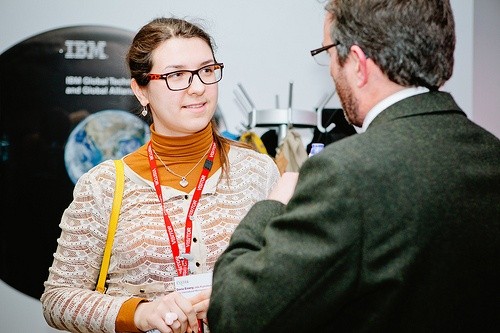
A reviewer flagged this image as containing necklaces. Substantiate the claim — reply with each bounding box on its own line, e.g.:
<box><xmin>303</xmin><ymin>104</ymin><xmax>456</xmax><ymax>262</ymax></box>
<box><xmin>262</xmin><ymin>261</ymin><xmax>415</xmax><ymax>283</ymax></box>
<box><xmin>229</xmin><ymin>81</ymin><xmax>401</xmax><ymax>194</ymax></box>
<box><xmin>152</xmin><ymin>141</ymin><xmax>213</xmax><ymax>188</ymax></box>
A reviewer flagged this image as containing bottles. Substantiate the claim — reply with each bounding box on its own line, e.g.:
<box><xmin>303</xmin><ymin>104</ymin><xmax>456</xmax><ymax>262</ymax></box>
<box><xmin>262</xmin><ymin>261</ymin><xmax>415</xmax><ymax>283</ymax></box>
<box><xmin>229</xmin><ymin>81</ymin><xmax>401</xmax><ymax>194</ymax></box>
<box><xmin>308</xmin><ymin>144</ymin><xmax>323</xmax><ymax>158</ymax></box>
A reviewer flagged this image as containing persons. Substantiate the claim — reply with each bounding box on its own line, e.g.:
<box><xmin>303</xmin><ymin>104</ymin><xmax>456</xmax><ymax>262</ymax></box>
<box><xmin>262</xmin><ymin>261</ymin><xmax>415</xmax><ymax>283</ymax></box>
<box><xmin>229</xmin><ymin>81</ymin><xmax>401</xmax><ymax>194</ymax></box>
<box><xmin>206</xmin><ymin>0</ymin><xmax>500</xmax><ymax>333</ymax></box>
<box><xmin>40</xmin><ymin>18</ymin><xmax>281</xmax><ymax>333</ymax></box>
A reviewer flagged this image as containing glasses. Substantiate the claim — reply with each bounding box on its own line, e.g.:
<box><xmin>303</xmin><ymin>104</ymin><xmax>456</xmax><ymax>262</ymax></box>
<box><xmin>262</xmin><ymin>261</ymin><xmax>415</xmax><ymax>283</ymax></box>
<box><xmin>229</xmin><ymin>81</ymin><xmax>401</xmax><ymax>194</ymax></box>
<box><xmin>148</xmin><ymin>62</ymin><xmax>224</xmax><ymax>92</ymax></box>
<box><xmin>310</xmin><ymin>42</ymin><xmax>340</xmax><ymax>68</ymax></box>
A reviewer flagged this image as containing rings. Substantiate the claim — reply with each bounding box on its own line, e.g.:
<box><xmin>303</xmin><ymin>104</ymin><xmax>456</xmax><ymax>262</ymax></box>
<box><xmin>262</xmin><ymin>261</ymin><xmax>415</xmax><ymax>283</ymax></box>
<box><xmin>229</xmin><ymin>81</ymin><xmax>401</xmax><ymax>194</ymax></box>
<box><xmin>165</xmin><ymin>312</ymin><xmax>178</xmax><ymax>325</ymax></box>
